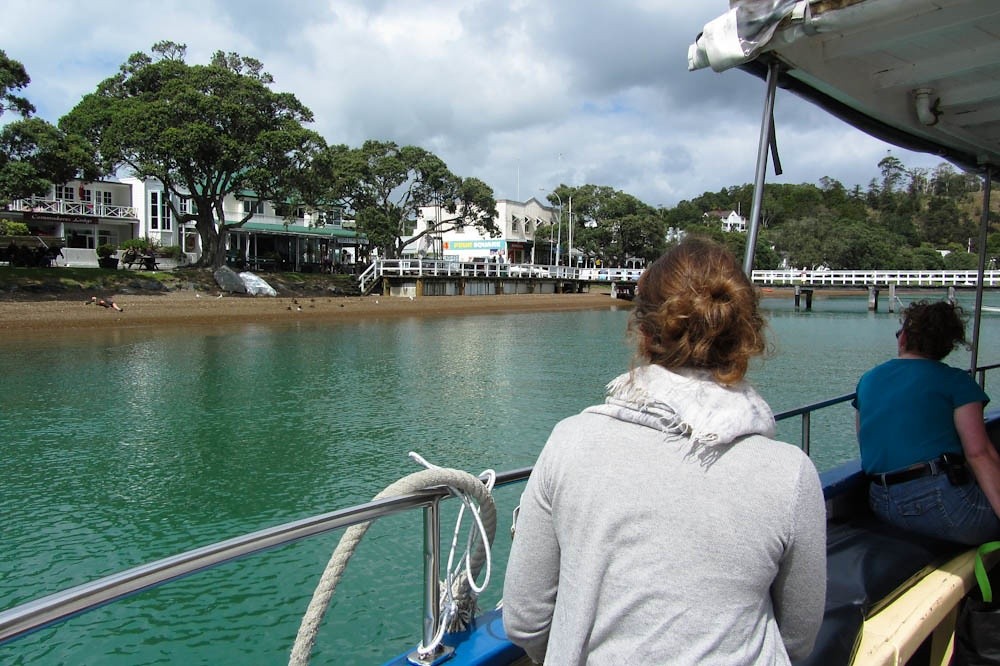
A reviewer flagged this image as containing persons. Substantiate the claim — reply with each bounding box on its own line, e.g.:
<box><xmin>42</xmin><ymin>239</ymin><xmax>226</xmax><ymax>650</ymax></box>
<box><xmin>121</xmin><ymin>245</ymin><xmax>158</xmax><ymax>271</ymax></box>
<box><xmin>500</xmin><ymin>238</ymin><xmax>827</xmax><ymax>665</ymax></box>
<box><xmin>340</xmin><ymin>246</ymin><xmax>648</xmax><ymax>286</ymax></box>
<box><xmin>850</xmin><ymin>296</ymin><xmax>1000</xmax><ymax>552</ymax></box>
<box><xmin>6</xmin><ymin>237</ymin><xmax>53</xmax><ymax>267</ymax></box>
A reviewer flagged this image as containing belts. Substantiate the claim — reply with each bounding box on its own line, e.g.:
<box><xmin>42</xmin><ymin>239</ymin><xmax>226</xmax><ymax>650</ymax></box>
<box><xmin>873</xmin><ymin>452</ymin><xmax>954</xmax><ymax>485</ymax></box>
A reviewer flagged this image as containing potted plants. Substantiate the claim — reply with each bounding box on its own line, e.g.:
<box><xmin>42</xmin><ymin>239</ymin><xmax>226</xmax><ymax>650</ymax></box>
<box><xmin>96</xmin><ymin>244</ymin><xmax>119</xmax><ymax>269</ymax></box>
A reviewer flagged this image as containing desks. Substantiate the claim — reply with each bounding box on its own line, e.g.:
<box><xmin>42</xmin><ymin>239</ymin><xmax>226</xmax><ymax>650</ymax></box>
<box><xmin>127</xmin><ymin>255</ymin><xmax>152</xmax><ymax>270</ymax></box>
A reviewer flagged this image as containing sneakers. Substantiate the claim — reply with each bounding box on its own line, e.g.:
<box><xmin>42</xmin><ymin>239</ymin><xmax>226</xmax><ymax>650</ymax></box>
<box><xmin>962</xmin><ymin>601</ymin><xmax>1000</xmax><ymax>660</ymax></box>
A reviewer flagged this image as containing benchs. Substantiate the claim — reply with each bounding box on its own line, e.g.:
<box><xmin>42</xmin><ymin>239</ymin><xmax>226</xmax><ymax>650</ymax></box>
<box><xmin>127</xmin><ymin>262</ymin><xmax>160</xmax><ymax>270</ymax></box>
<box><xmin>850</xmin><ymin>548</ymin><xmax>1000</xmax><ymax>666</ymax></box>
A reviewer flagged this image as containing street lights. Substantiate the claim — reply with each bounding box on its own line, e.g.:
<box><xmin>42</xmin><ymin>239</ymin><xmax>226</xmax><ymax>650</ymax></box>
<box><xmin>539</xmin><ymin>188</ymin><xmax>561</xmax><ymax>266</ymax></box>
